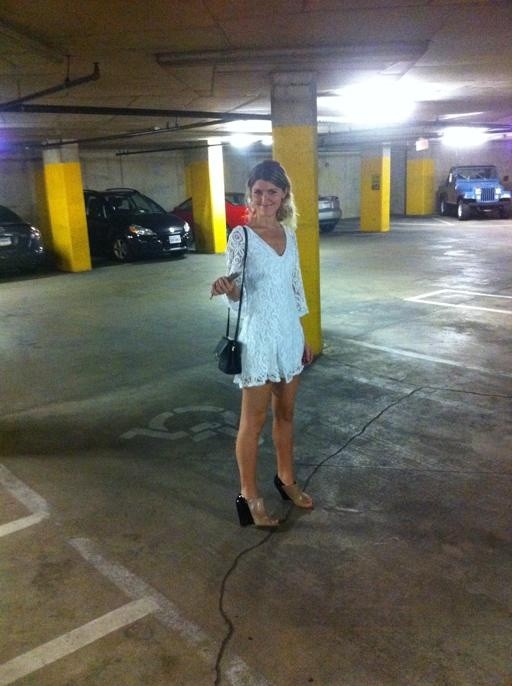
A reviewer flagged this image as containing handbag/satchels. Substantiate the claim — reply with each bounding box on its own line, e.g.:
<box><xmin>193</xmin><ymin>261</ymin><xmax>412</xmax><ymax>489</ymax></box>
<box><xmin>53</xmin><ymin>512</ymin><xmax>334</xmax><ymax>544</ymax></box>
<box><xmin>213</xmin><ymin>336</ymin><xmax>242</xmax><ymax>375</ymax></box>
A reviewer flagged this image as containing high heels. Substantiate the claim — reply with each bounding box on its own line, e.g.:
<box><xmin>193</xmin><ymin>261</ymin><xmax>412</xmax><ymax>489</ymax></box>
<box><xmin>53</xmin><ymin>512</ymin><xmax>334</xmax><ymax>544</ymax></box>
<box><xmin>236</xmin><ymin>493</ymin><xmax>282</xmax><ymax>528</ymax></box>
<box><xmin>274</xmin><ymin>473</ymin><xmax>314</xmax><ymax>509</ymax></box>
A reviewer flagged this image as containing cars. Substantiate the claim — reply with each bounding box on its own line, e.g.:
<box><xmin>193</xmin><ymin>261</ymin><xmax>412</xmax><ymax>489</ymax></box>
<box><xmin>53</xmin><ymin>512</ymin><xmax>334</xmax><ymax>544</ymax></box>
<box><xmin>317</xmin><ymin>193</ymin><xmax>341</xmax><ymax>232</ymax></box>
<box><xmin>167</xmin><ymin>190</ymin><xmax>254</xmax><ymax>238</ymax></box>
<box><xmin>0</xmin><ymin>204</ymin><xmax>47</xmax><ymax>275</ymax></box>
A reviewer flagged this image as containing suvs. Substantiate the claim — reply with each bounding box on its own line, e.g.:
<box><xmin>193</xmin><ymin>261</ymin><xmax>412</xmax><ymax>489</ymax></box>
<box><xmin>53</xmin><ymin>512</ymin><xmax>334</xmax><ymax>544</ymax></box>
<box><xmin>438</xmin><ymin>164</ymin><xmax>512</xmax><ymax>222</ymax></box>
<box><xmin>84</xmin><ymin>187</ymin><xmax>195</xmax><ymax>263</ymax></box>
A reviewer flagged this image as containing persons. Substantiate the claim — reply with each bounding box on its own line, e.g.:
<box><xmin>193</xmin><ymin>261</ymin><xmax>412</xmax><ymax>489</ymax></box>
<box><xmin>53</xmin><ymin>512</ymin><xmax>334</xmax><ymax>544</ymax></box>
<box><xmin>209</xmin><ymin>157</ymin><xmax>318</xmax><ymax>530</ymax></box>
